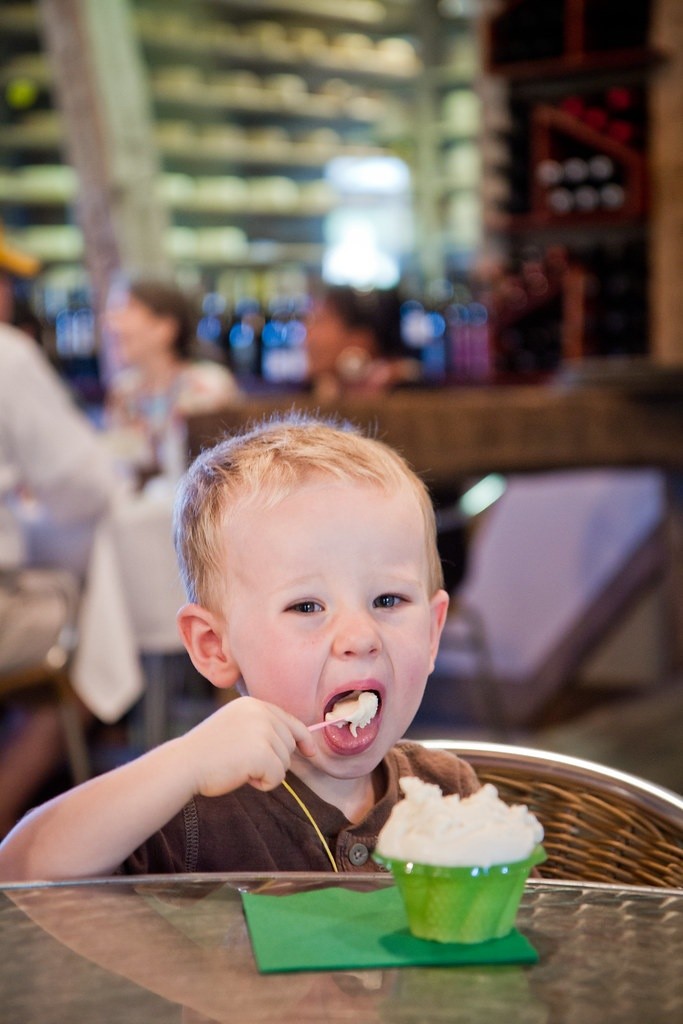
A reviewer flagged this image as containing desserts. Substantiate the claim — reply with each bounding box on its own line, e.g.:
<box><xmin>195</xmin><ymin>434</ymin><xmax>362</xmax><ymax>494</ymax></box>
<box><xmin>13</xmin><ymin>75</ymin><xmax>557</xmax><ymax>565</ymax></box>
<box><xmin>375</xmin><ymin>777</ymin><xmax>546</xmax><ymax>942</ymax></box>
<box><xmin>325</xmin><ymin>690</ymin><xmax>378</xmax><ymax>737</ymax></box>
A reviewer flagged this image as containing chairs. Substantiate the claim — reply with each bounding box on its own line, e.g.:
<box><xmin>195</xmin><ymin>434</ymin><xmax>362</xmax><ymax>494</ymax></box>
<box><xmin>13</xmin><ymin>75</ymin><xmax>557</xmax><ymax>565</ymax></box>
<box><xmin>411</xmin><ymin>736</ymin><xmax>683</xmax><ymax>889</ymax></box>
<box><xmin>420</xmin><ymin>471</ymin><xmax>669</xmax><ymax>727</ymax></box>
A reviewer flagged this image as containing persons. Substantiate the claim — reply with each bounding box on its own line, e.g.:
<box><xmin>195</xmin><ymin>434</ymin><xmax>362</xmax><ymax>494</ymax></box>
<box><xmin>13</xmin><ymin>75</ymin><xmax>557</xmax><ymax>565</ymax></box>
<box><xmin>0</xmin><ymin>232</ymin><xmax>475</xmax><ymax>828</ymax></box>
<box><xmin>3</xmin><ymin>408</ymin><xmax>547</xmax><ymax>880</ymax></box>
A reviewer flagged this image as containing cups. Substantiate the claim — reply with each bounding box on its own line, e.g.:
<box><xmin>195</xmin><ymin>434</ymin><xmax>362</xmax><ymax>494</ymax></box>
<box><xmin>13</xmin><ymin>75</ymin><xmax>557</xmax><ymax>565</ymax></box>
<box><xmin>372</xmin><ymin>841</ymin><xmax>546</xmax><ymax>946</ymax></box>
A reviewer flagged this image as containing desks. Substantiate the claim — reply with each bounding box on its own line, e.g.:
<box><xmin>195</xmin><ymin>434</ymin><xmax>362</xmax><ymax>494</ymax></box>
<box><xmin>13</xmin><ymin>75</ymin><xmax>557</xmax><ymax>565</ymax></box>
<box><xmin>0</xmin><ymin>871</ymin><xmax>683</xmax><ymax>1024</ymax></box>
